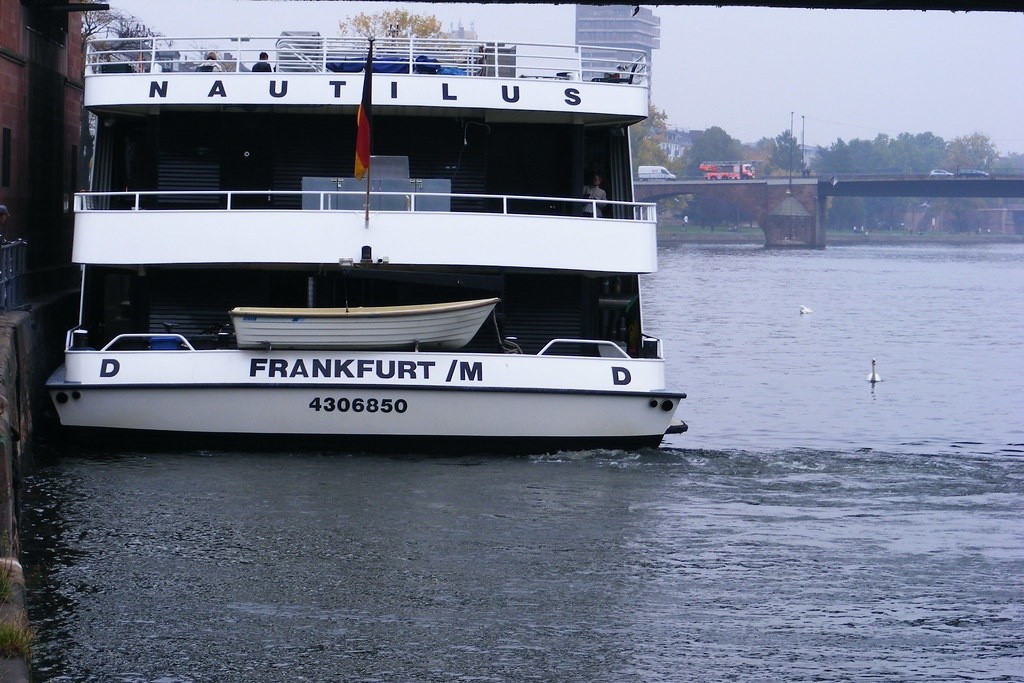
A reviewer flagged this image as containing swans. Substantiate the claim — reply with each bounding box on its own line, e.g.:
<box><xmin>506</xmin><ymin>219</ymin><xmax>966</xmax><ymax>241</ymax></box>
<box><xmin>867</xmin><ymin>358</ymin><xmax>881</xmax><ymax>381</ymax></box>
<box><xmin>799</xmin><ymin>305</ymin><xmax>812</xmax><ymax>313</ymax></box>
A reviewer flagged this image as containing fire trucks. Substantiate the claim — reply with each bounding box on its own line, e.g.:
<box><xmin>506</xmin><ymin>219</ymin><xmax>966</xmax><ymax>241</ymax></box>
<box><xmin>698</xmin><ymin>159</ymin><xmax>764</xmax><ymax>180</ymax></box>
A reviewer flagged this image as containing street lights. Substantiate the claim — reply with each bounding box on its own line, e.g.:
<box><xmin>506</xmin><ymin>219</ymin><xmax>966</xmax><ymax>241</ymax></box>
<box><xmin>801</xmin><ymin>115</ymin><xmax>805</xmax><ymax>178</ymax></box>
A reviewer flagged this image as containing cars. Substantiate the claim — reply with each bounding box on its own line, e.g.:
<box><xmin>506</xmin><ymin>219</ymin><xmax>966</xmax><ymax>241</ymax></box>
<box><xmin>955</xmin><ymin>169</ymin><xmax>989</xmax><ymax>178</ymax></box>
<box><xmin>929</xmin><ymin>169</ymin><xmax>954</xmax><ymax>178</ymax></box>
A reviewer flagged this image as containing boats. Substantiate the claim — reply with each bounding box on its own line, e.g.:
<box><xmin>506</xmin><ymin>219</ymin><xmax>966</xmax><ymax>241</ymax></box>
<box><xmin>41</xmin><ymin>38</ymin><xmax>690</xmax><ymax>452</ymax></box>
<box><xmin>226</xmin><ymin>296</ymin><xmax>503</xmax><ymax>350</ymax></box>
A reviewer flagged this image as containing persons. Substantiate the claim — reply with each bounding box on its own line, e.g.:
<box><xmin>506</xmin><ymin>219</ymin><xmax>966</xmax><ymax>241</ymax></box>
<box><xmin>201</xmin><ymin>52</ymin><xmax>222</xmax><ymax>72</ymax></box>
<box><xmin>153</xmin><ymin>63</ymin><xmax>162</xmax><ymax>72</ymax></box>
<box><xmin>582</xmin><ymin>174</ymin><xmax>607</xmax><ymax>217</ymax></box>
<box><xmin>354</xmin><ymin>45</ymin><xmax>372</xmax><ymax>181</ymax></box>
<box><xmin>252</xmin><ymin>52</ymin><xmax>272</xmax><ymax>72</ymax></box>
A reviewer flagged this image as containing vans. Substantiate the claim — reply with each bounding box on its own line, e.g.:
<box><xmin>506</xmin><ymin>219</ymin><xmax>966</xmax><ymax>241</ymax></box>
<box><xmin>637</xmin><ymin>165</ymin><xmax>676</xmax><ymax>181</ymax></box>
